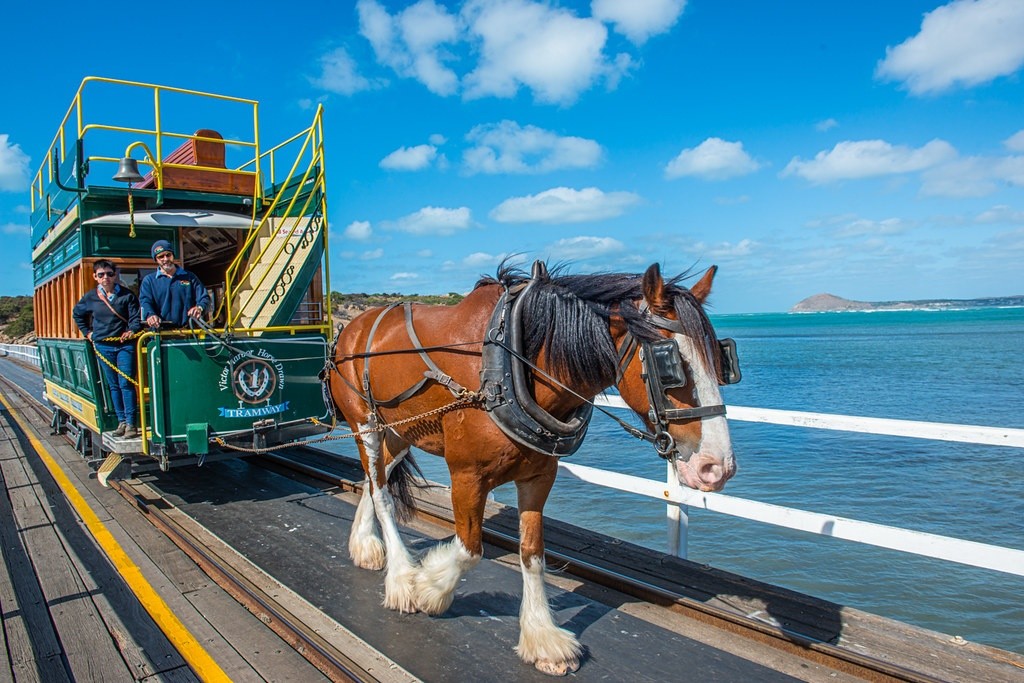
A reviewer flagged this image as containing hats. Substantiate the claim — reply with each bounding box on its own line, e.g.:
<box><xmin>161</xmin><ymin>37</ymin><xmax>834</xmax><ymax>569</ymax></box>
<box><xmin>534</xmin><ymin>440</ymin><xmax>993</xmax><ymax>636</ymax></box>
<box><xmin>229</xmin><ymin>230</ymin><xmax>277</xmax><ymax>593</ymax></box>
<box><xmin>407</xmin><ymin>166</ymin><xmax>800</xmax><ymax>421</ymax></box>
<box><xmin>150</xmin><ymin>239</ymin><xmax>174</xmax><ymax>262</ymax></box>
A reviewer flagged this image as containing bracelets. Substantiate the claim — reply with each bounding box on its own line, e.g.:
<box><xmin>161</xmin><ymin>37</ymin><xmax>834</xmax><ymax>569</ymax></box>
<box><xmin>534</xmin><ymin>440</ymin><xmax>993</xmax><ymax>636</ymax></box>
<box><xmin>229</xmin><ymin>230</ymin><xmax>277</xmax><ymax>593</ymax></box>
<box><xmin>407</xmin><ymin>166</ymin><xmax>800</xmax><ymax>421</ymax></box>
<box><xmin>198</xmin><ymin>306</ymin><xmax>203</xmax><ymax>311</ymax></box>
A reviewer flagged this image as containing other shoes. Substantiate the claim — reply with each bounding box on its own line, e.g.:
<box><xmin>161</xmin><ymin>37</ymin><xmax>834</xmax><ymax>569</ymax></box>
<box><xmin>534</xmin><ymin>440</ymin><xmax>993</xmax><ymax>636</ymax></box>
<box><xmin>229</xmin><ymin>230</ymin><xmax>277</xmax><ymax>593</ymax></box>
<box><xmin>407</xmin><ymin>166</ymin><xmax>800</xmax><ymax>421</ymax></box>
<box><xmin>122</xmin><ymin>425</ymin><xmax>137</xmax><ymax>439</ymax></box>
<box><xmin>112</xmin><ymin>422</ymin><xmax>126</xmax><ymax>437</ymax></box>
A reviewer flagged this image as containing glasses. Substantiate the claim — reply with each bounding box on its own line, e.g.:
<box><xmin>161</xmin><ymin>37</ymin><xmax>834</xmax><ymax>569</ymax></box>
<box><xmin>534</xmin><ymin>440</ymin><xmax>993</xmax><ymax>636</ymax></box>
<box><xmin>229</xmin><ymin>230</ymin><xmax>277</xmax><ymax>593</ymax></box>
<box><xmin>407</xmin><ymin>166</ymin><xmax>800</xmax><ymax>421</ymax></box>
<box><xmin>156</xmin><ymin>252</ymin><xmax>172</xmax><ymax>260</ymax></box>
<box><xmin>95</xmin><ymin>272</ymin><xmax>115</xmax><ymax>278</ymax></box>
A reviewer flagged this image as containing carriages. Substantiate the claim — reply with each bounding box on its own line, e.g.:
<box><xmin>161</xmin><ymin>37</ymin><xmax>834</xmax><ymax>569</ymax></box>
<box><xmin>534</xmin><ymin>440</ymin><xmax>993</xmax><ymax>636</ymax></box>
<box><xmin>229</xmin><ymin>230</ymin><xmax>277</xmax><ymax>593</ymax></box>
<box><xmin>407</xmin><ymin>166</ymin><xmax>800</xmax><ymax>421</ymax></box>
<box><xmin>27</xmin><ymin>74</ymin><xmax>741</xmax><ymax>678</ymax></box>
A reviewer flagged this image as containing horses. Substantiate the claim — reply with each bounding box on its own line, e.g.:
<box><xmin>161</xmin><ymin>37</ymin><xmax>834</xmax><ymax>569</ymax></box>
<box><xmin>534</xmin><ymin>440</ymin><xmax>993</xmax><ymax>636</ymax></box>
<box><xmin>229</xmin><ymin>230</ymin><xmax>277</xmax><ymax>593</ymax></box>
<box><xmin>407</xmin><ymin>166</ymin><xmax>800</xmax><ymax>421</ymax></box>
<box><xmin>328</xmin><ymin>264</ymin><xmax>737</xmax><ymax>678</ymax></box>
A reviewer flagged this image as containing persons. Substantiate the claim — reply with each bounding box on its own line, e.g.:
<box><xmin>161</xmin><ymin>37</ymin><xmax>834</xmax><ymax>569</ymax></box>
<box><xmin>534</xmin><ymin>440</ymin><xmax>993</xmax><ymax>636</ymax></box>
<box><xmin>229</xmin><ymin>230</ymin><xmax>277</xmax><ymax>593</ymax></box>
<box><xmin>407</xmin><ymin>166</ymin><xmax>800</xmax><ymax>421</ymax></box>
<box><xmin>139</xmin><ymin>239</ymin><xmax>212</xmax><ymax>339</ymax></box>
<box><xmin>72</xmin><ymin>259</ymin><xmax>141</xmax><ymax>439</ymax></box>
